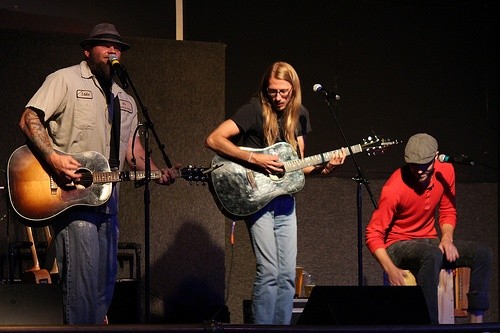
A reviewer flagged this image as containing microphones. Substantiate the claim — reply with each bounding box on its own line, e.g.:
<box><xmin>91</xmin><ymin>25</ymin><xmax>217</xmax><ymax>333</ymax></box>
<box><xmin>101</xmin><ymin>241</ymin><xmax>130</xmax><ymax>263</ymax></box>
<box><xmin>312</xmin><ymin>84</ymin><xmax>340</xmax><ymax>101</ymax></box>
<box><xmin>438</xmin><ymin>154</ymin><xmax>475</xmax><ymax>166</ymax></box>
<box><xmin>110</xmin><ymin>54</ymin><xmax>129</xmax><ymax>88</ymax></box>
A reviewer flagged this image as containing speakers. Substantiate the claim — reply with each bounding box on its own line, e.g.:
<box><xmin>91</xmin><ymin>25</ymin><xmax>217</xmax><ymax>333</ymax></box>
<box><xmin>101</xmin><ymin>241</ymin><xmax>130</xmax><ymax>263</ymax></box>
<box><xmin>297</xmin><ymin>285</ymin><xmax>431</xmax><ymax>327</ymax></box>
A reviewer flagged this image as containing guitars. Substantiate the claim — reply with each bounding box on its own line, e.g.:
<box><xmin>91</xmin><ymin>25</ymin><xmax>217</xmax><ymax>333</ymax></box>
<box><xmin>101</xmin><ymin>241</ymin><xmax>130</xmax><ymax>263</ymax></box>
<box><xmin>211</xmin><ymin>132</ymin><xmax>397</xmax><ymax>217</ymax></box>
<box><xmin>15</xmin><ymin>225</ymin><xmax>60</xmax><ymax>283</ymax></box>
<box><xmin>7</xmin><ymin>142</ymin><xmax>211</xmax><ymax>222</ymax></box>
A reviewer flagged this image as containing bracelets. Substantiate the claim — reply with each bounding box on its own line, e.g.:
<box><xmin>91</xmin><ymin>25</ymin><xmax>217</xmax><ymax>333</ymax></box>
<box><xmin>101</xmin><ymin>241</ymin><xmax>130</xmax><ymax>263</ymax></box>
<box><xmin>248</xmin><ymin>152</ymin><xmax>254</xmax><ymax>163</ymax></box>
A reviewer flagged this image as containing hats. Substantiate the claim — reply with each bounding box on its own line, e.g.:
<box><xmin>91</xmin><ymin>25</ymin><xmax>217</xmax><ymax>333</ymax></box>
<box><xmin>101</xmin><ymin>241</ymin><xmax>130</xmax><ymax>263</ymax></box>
<box><xmin>404</xmin><ymin>134</ymin><xmax>438</xmax><ymax>165</ymax></box>
<box><xmin>81</xmin><ymin>24</ymin><xmax>131</xmax><ymax>52</ymax></box>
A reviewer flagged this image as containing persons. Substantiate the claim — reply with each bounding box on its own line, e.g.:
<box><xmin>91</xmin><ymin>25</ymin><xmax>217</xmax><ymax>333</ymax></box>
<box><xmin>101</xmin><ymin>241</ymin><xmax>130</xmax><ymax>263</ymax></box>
<box><xmin>18</xmin><ymin>23</ymin><xmax>182</xmax><ymax>325</ymax></box>
<box><xmin>206</xmin><ymin>61</ymin><xmax>346</xmax><ymax>326</ymax></box>
<box><xmin>366</xmin><ymin>132</ymin><xmax>493</xmax><ymax>324</ymax></box>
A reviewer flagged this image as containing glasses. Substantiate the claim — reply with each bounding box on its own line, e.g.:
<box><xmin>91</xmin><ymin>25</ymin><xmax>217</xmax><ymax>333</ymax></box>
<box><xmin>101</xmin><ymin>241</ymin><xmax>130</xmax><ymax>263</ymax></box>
<box><xmin>267</xmin><ymin>86</ymin><xmax>293</xmax><ymax>99</ymax></box>
